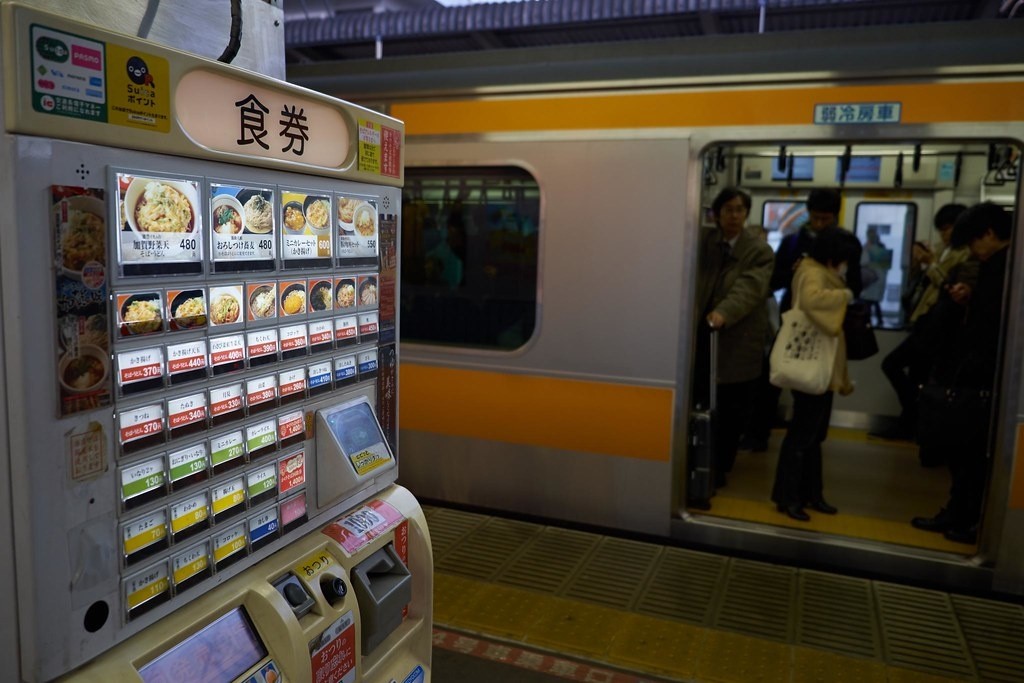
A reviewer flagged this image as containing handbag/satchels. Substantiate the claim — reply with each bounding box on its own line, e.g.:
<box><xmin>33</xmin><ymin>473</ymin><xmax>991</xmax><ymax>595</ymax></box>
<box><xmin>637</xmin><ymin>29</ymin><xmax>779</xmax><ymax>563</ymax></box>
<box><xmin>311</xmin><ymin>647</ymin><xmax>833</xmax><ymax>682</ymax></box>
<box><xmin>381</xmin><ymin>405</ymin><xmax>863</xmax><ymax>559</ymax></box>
<box><xmin>768</xmin><ymin>267</ymin><xmax>839</xmax><ymax>395</ymax></box>
<box><xmin>841</xmin><ymin>300</ymin><xmax>878</xmax><ymax>360</ymax></box>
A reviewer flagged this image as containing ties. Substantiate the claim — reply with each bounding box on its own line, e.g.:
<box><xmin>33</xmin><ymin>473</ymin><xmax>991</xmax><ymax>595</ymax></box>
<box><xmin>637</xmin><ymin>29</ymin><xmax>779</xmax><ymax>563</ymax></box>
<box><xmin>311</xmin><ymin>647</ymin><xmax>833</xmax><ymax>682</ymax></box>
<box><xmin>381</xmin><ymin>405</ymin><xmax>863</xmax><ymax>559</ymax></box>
<box><xmin>719</xmin><ymin>242</ymin><xmax>730</xmax><ymax>273</ymax></box>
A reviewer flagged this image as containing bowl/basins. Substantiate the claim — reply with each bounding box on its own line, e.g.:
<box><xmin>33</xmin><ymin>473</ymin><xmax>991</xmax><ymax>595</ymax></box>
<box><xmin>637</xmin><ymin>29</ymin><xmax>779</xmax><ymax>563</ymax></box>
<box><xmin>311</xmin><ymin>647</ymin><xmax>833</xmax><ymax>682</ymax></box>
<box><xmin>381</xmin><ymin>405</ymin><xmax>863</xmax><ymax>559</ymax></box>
<box><xmin>120</xmin><ymin>293</ymin><xmax>162</xmax><ymax>334</ymax></box>
<box><xmin>353</xmin><ymin>203</ymin><xmax>376</xmax><ymax>238</ymax></box>
<box><xmin>57</xmin><ymin>343</ymin><xmax>110</xmax><ymax>392</ymax></box>
<box><xmin>338</xmin><ymin>200</ymin><xmax>368</xmax><ymax>231</ymax></box>
<box><xmin>358</xmin><ymin>277</ymin><xmax>377</xmax><ymax>305</ymax></box>
<box><xmin>123</xmin><ymin>177</ymin><xmax>199</xmax><ymax>243</ymax></box>
<box><xmin>169</xmin><ymin>289</ymin><xmax>206</xmax><ymax>330</ymax></box>
<box><xmin>308</xmin><ymin>280</ymin><xmax>331</xmax><ymax>312</ymax></box>
<box><xmin>248</xmin><ymin>286</ymin><xmax>275</xmax><ymax>319</ymax></box>
<box><xmin>334</xmin><ymin>279</ymin><xmax>355</xmax><ymax>309</ymax></box>
<box><xmin>51</xmin><ymin>193</ymin><xmax>105</xmax><ymax>281</ymax></box>
<box><xmin>282</xmin><ymin>200</ymin><xmax>306</xmax><ymax>235</ymax></box>
<box><xmin>209</xmin><ymin>287</ymin><xmax>242</xmax><ymax>326</ymax></box>
<box><xmin>211</xmin><ymin>193</ymin><xmax>245</xmax><ymax>242</ymax></box>
<box><xmin>302</xmin><ymin>195</ymin><xmax>330</xmax><ymax>236</ymax></box>
<box><xmin>280</xmin><ymin>283</ymin><xmax>305</xmax><ymax>316</ymax></box>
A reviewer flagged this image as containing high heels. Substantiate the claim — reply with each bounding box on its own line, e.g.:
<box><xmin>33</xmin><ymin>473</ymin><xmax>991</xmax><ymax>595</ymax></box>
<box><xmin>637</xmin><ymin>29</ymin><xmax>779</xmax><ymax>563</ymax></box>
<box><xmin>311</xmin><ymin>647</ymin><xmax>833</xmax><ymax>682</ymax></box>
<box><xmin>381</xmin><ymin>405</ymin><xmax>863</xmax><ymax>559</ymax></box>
<box><xmin>803</xmin><ymin>495</ymin><xmax>837</xmax><ymax>514</ymax></box>
<box><xmin>777</xmin><ymin>503</ymin><xmax>810</xmax><ymax>521</ymax></box>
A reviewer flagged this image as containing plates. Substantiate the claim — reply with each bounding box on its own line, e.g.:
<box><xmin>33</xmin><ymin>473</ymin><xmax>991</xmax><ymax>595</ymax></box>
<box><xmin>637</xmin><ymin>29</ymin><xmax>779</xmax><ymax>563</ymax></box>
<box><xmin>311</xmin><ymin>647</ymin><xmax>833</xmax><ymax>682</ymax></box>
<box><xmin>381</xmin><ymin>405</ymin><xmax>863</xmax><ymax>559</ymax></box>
<box><xmin>246</xmin><ymin>202</ymin><xmax>272</xmax><ymax>233</ymax></box>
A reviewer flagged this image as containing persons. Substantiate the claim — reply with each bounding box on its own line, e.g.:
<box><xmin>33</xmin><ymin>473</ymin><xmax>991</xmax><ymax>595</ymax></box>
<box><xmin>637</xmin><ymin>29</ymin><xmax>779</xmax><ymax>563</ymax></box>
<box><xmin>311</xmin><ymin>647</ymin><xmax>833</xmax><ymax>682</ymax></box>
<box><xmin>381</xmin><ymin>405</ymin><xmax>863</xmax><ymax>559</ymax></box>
<box><xmin>704</xmin><ymin>184</ymin><xmax>1012</xmax><ymax>546</ymax></box>
<box><xmin>400</xmin><ymin>198</ymin><xmax>536</xmax><ymax>351</ymax></box>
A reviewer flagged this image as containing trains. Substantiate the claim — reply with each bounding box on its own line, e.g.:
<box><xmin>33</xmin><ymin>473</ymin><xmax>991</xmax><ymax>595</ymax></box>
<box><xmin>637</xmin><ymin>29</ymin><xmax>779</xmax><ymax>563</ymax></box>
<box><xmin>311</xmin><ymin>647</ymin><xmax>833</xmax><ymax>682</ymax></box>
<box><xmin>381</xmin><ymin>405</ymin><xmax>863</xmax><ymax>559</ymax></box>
<box><xmin>284</xmin><ymin>20</ymin><xmax>1023</xmax><ymax>606</ymax></box>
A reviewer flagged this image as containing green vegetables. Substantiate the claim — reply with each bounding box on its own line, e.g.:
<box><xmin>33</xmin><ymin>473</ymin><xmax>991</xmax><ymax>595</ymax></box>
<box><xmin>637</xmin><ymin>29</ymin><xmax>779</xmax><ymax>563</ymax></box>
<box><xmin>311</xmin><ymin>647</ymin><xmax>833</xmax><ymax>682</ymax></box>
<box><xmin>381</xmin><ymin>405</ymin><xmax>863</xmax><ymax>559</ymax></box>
<box><xmin>218</xmin><ymin>209</ymin><xmax>234</xmax><ymax>224</ymax></box>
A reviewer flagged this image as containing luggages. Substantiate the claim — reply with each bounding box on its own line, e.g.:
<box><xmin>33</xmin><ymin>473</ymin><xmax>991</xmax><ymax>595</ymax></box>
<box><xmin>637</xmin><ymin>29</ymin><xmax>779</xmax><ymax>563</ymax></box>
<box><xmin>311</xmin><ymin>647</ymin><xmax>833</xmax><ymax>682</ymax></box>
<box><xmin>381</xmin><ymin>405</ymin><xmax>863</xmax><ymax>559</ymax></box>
<box><xmin>684</xmin><ymin>318</ymin><xmax>720</xmax><ymax>510</ymax></box>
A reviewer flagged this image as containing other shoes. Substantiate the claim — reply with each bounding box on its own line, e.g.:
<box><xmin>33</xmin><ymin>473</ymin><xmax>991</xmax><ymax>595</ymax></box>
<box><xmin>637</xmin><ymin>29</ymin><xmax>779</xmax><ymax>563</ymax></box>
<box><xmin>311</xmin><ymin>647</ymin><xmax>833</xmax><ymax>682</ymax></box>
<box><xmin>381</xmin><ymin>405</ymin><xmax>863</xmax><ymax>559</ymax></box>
<box><xmin>911</xmin><ymin>507</ymin><xmax>976</xmax><ymax>532</ymax></box>
<box><xmin>944</xmin><ymin>519</ymin><xmax>977</xmax><ymax>545</ymax></box>
<box><xmin>714</xmin><ymin>473</ymin><xmax>724</xmax><ymax>487</ymax></box>
